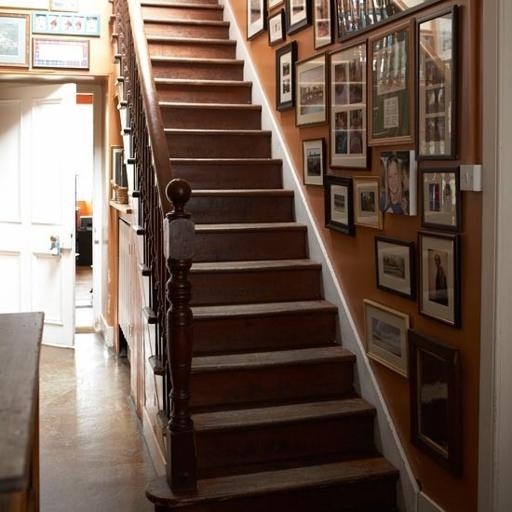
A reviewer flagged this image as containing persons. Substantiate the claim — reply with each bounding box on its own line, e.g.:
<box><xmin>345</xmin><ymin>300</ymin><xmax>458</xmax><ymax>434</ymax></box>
<box><xmin>379</xmin><ymin>155</ymin><xmax>410</xmax><ymax>215</ymax></box>
<box><xmin>398</xmin><ymin>160</ymin><xmax>409</xmax><ymax>203</ymax></box>
<box><xmin>433</xmin><ymin>254</ymin><xmax>448</xmax><ymax>305</ymax></box>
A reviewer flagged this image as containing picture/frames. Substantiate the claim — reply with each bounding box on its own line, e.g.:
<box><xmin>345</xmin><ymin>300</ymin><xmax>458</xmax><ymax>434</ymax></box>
<box><xmin>361</xmin><ymin>296</ymin><xmax>412</xmax><ymax>380</ymax></box>
<box><xmin>247</xmin><ymin>4</ymin><xmax>461</xmax><ymax>236</ymax></box>
<box><xmin>408</xmin><ymin>326</ymin><xmax>463</xmax><ymax>472</ymax></box>
<box><xmin>415</xmin><ymin>229</ymin><xmax>461</xmax><ymax>329</ymax></box>
<box><xmin>30</xmin><ymin>38</ymin><xmax>91</xmax><ymax>71</ymax></box>
<box><xmin>371</xmin><ymin>232</ymin><xmax>417</xmax><ymax>303</ymax></box>
<box><xmin>0</xmin><ymin>12</ymin><xmax>31</xmax><ymax>68</ymax></box>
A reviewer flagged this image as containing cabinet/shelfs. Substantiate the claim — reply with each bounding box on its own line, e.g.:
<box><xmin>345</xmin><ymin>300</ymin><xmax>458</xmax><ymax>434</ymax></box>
<box><xmin>110</xmin><ymin>199</ymin><xmax>135</xmax><ymax>361</ymax></box>
